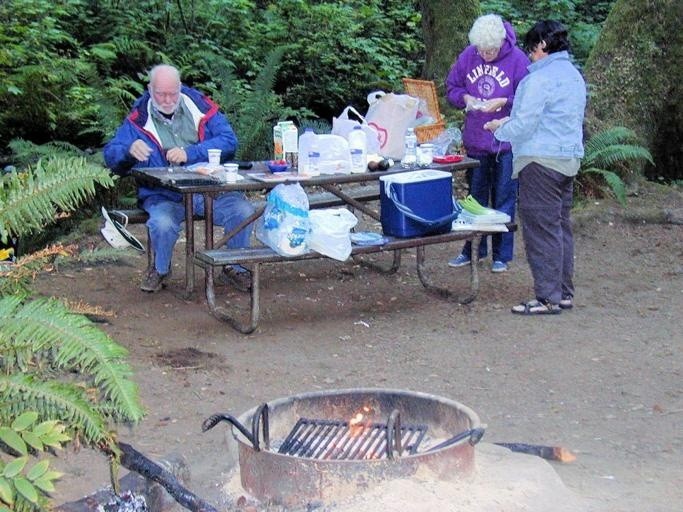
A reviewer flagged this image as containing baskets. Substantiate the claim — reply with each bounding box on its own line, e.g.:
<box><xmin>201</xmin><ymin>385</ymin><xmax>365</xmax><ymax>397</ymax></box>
<box><xmin>403</xmin><ymin>77</ymin><xmax>448</xmax><ymax>144</ymax></box>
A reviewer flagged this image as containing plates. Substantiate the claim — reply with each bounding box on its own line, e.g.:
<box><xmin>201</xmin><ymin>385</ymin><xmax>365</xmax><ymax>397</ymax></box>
<box><xmin>433</xmin><ymin>155</ymin><xmax>463</xmax><ymax>164</ymax></box>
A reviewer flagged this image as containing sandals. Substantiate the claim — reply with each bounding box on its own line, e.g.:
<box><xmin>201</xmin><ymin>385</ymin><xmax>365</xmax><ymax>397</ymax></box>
<box><xmin>512</xmin><ymin>297</ymin><xmax>562</xmax><ymax>314</ymax></box>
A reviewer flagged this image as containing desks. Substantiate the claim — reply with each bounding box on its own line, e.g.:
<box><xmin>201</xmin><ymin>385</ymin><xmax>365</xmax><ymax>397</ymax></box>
<box><xmin>131</xmin><ymin>146</ymin><xmax>481</xmax><ymax>308</ymax></box>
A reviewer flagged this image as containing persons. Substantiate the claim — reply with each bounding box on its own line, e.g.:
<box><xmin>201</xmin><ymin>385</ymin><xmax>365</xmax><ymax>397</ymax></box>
<box><xmin>483</xmin><ymin>21</ymin><xmax>576</xmax><ymax>314</ymax></box>
<box><xmin>103</xmin><ymin>64</ymin><xmax>253</xmax><ymax>294</ymax></box>
<box><xmin>444</xmin><ymin>12</ymin><xmax>531</xmax><ymax>273</ymax></box>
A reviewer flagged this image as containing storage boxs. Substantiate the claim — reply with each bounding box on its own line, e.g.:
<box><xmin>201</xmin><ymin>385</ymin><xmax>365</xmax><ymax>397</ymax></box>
<box><xmin>380</xmin><ymin>170</ymin><xmax>463</xmax><ymax>238</ymax></box>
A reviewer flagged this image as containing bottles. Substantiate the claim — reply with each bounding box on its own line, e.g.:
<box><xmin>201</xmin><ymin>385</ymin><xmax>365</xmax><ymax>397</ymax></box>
<box><xmin>298</xmin><ymin>129</ymin><xmax>319</xmax><ymax>178</ymax></box>
<box><xmin>401</xmin><ymin>127</ymin><xmax>416</xmax><ymax>167</ymax></box>
<box><xmin>347</xmin><ymin>125</ymin><xmax>367</xmax><ymax>174</ymax></box>
<box><xmin>416</xmin><ymin>143</ymin><xmax>435</xmax><ymax>167</ymax></box>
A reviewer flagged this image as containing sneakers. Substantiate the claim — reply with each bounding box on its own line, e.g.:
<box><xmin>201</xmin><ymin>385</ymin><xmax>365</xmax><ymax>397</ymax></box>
<box><xmin>492</xmin><ymin>259</ymin><xmax>508</xmax><ymax>272</ymax></box>
<box><xmin>219</xmin><ymin>265</ymin><xmax>252</xmax><ymax>291</ymax></box>
<box><xmin>140</xmin><ymin>267</ymin><xmax>172</xmax><ymax>291</ymax></box>
<box><xmin>448</xmin><ymin>254</ymin><xmax>472</xmax><ymax>267</ymax></box>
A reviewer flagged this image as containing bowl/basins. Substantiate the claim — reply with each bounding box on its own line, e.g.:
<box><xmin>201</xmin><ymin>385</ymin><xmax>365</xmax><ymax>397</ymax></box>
<box><xmin>263</xmin><ymin>159</ymin><xmax>290</xmax><ymax>175</ymax></box>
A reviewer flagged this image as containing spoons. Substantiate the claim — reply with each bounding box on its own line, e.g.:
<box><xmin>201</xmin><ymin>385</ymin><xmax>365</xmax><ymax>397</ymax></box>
<box><xmin>434</xmin><ymin>156</ymin><xmax>464</xmax><ymax>159</ymax></box>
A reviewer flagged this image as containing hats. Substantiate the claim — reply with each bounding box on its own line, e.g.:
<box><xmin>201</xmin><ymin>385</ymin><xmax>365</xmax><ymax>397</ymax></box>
<box><xmin>100</xmin><ymin>206</ymin><xmax>145</xmax><ymax>251</ymax></box>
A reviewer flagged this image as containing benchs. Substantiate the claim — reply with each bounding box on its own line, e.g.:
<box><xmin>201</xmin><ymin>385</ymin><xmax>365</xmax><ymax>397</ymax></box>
<box><xmin>191</xmin><ymin>221</ymin><xmax>522</xmax><ymax>336</ymax></box>
<box><xmin>105</xmin><ymin>186</ymin><xmax>388</xmax><ymax>278</ymax></box>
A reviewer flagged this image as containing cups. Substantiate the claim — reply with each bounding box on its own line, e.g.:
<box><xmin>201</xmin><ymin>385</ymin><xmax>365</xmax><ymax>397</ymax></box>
<box><xmin>284</xmin><ymin>151</ymin><xmax>298</xmax><ymax>170</ymax></box>
<box><xmin>208</xmin><ymin>149</ymin><xmax>239</xmax><ymax>185</ymax></box>
